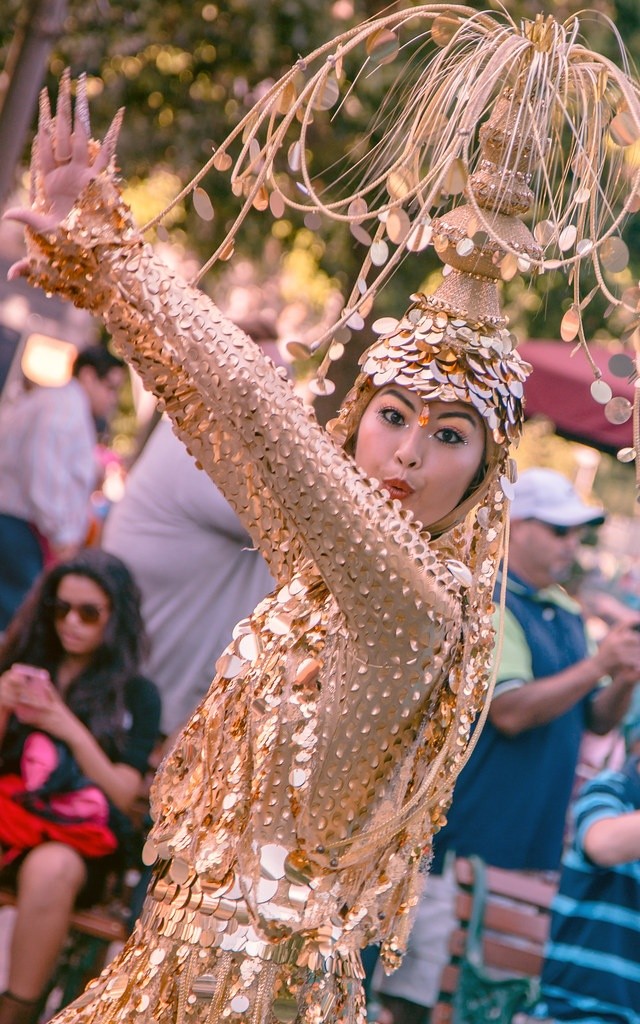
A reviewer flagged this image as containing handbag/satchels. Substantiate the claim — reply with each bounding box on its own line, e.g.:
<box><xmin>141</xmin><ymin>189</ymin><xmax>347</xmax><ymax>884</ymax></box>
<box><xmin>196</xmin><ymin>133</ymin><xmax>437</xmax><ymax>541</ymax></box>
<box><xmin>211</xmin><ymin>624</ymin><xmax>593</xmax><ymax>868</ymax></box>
<box><xmin>1</xmin><ymin>778</ymin><xmax>116</xmax><ymax>868</ymax></box>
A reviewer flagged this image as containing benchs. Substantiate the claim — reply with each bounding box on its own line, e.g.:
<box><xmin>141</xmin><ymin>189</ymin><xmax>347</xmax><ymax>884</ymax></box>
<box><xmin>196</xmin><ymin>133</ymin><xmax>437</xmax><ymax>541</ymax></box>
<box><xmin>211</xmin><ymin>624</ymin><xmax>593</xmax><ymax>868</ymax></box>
<box><xmin>0</xmin><ymin>743</ymin><xmax>174</xmax><ymax>1013</ymax></box>
<box><xmin>430</xmin><ymin>859</ymin><xmax>554</xmax><ymax>1024</ymax></box>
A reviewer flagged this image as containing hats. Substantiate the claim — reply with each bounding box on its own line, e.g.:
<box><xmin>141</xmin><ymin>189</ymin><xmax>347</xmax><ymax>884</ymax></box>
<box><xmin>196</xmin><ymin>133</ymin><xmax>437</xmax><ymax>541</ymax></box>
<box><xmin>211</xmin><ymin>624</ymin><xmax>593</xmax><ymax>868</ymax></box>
<box><xmin>510</xmin><ymin>469</ymin><xmax>606</xmax><ymax>528</ymax></box>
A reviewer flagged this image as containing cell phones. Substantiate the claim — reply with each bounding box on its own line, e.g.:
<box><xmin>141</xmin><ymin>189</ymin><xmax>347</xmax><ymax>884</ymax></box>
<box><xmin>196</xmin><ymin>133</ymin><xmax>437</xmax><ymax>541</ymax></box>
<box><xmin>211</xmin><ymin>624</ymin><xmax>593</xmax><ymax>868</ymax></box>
<box><xmin>12</xmin><ymin>664</ymin><xmax>49</xmax><ymax>723</ymax></box>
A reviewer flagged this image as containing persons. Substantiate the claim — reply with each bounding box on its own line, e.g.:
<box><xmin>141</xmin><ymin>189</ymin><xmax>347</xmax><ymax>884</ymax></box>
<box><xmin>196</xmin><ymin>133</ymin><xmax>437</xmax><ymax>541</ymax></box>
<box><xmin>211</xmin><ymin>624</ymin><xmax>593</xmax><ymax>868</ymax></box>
<box><xmin>2</xmin><ymin>342</ymin><xmax>132</xmax><ymax>631</ymax></box>
<box><xmin>97</xmin><ymin>312</ymin><xmax>291</xmax><ymax>766</ymax></box>
<box><xmin>1</xmin><ymin>549</ymin><xmax>166</xmax><ymax>1022</ymax></box>
<box><xmin>366</xmin><ymin>466</ymin><xmax>640</xmax><ymax>1024</ymax></box>
<box><xmin>4</xmin><ymin>66</ymin><xmax>538</xmax><ymax>1020</ymax></box>
<box><xmin>527</xmin><ymin>742</ymin><xmax>639</xmax><ymax>1024</ymax></box>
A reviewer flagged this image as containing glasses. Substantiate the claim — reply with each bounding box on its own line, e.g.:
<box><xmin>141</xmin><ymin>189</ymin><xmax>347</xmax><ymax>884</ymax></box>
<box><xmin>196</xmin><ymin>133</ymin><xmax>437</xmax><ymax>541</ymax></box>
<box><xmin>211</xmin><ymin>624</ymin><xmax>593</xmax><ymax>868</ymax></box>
<box><xmin>52</xmin><ymin>599</ymin><xmax>106</xmax><ymax>624</ymax></box>
